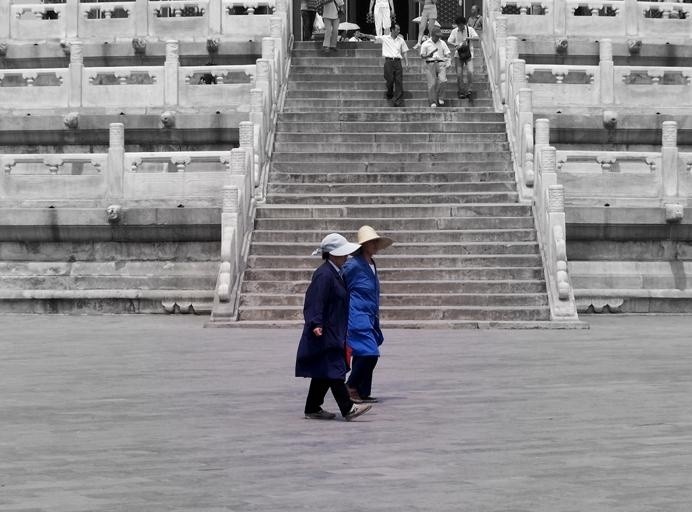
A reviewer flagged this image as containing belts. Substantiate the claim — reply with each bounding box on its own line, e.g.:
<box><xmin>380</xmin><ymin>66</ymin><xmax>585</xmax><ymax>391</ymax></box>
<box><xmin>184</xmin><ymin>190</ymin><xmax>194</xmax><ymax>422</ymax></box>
<box><xmin>426</xmin><ymin>60</ymin><xmax>443</xmax><ymax>63</ymax></box>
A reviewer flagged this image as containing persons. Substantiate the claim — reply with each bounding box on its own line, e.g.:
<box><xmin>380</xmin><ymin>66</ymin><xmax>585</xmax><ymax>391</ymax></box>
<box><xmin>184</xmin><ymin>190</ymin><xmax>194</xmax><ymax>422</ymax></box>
<box><xmin>447</xmin><ymin>17</ymin><xmax>480</xmax><ymax>98</ymax></box>
<box><xmin>358</xmin><ymin>23</ymin><xmax>411</xmax><ymax>106</ymax></box>
<box><xmin>420</xmin><ymin>27</ymin><xmax>451</xmax><ymax>107</ymax></box>
<box><xmin>343</xmin><ymin>224</ymin><xmax>393</xmax><ymax>403</ymax></box>
<box><xmin>293</xmin><ymin>232</ymin><xmax>373</xmax><ymax>424</ymax></box>
<box><xmin>300</xmin><ymin>0</ymin><xmax>484</xmax><ymax>45</ymax></box>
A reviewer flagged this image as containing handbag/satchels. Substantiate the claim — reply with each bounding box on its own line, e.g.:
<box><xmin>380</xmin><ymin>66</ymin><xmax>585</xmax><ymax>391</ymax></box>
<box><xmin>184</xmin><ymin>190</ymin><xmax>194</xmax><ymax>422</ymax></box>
<box><xmin>458</xmin><ymin>46</ymin><xmax>471</xmax><ymax>60</ymax></box>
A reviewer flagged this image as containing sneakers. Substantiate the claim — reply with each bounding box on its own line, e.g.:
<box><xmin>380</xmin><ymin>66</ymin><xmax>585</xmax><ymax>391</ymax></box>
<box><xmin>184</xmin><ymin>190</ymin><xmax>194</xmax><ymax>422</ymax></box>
<box><xmin>431</xmin><ymin>99</ymin><xmax>444</xmax><ymax>107</ymax></box>
<box><xmin>305</xmin><ymin>410</ymin><xmax>335</xmax><ymax>419</ymax></box>
<box><xmin>345</xmin><ymin>383</ymin><xmax>377</xmax><ymax>421</ymax></box>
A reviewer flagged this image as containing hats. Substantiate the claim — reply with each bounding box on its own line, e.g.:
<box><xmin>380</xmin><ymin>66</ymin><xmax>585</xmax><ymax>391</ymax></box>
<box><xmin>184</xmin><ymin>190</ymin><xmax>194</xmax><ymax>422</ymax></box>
<box><xmin>321</xmin><ymin>226</ymin><xmax>392</xmax><ymax>256</ymax></box>
